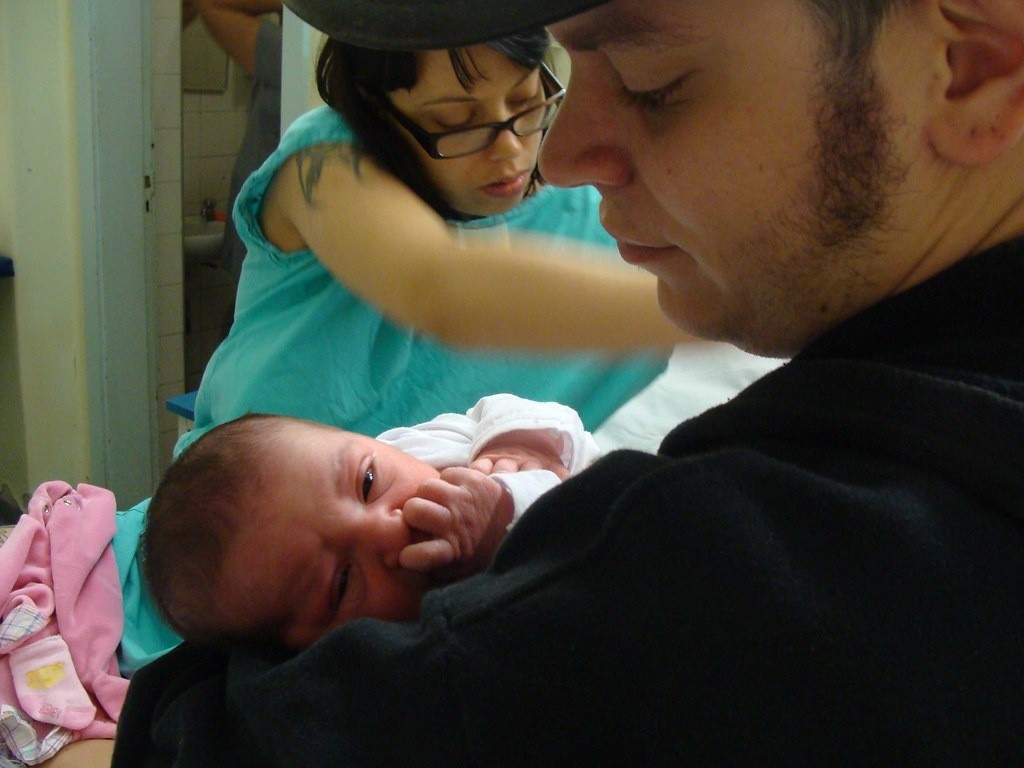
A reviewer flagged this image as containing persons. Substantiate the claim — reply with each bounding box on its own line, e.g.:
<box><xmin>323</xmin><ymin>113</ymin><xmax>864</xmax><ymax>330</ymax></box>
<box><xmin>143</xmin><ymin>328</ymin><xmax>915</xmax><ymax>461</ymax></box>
<box><xmin>147</xmin><ymin>394</ymin><xmax>597</xmax><ymax>658</ymax></box>
<box><xmin>182</xmin><ymin>0</ymin><xmax>200</xmax><ymax>29</ymax></box>
<box><xmin>0</xmin><ymin>0</ymin><xmax>1024</xmax><ymax>768</ymax></box>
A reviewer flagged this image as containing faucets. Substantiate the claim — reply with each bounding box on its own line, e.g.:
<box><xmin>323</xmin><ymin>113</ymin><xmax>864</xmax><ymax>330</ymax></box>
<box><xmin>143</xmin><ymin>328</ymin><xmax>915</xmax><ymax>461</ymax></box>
<box><xmin>199</xmin><ymin>198</ymin><xmax>216</xmax><ymax>222</ymax></box>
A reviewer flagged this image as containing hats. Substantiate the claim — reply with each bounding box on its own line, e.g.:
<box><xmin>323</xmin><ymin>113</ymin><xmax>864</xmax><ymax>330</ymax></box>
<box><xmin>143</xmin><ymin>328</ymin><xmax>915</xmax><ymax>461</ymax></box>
<box><xmin>287</xmin><ymin>0</ymin><xmax>606</xmax><ymax>52</ymax></box>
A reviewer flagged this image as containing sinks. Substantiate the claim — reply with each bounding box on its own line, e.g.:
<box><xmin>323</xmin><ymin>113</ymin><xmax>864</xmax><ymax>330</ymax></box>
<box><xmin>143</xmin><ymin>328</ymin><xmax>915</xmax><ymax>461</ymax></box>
<box><xmin>178</xmin><ymin>216</ymin><xmax>225</xmax><ymax>265</ymax></box>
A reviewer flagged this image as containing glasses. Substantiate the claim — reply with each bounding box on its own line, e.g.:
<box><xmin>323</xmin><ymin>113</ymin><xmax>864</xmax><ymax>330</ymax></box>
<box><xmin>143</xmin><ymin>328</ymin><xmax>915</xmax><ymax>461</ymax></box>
<box><xmin>364</xmin><ymin>60</ymin><xmax>568</xmax><ymax>161</ymax></box>
